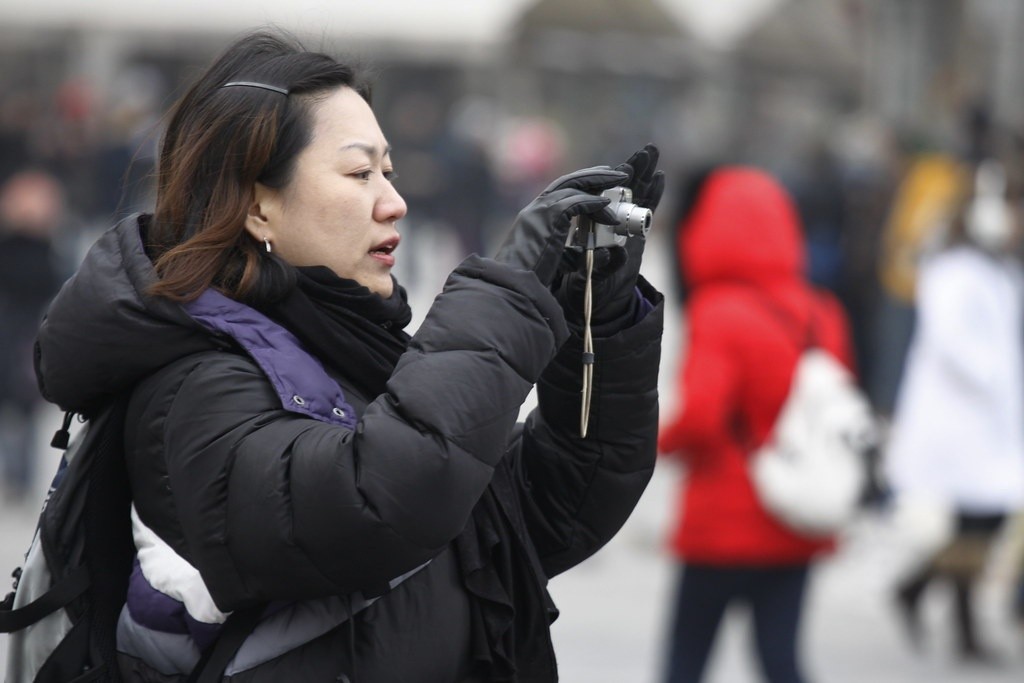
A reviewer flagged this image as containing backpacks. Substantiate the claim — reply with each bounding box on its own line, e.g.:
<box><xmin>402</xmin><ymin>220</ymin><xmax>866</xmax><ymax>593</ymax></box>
<box><xmin>0</xmin><ymin>391</ymin><xmax>262</xmax><ymax>683</ymax></box>
<box><xmin>729</xmin><ymin>280</ymin><xmax>879</xmax><ymax>535</ymax></box>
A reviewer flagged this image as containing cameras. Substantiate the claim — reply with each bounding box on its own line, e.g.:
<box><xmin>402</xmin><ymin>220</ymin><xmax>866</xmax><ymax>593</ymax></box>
<box><xmin>576</xmin><ymin>186</ymin><xmax>653</xmax><ymax>251</ymax></box>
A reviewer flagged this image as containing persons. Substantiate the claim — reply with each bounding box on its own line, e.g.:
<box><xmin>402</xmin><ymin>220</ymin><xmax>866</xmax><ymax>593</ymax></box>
<box><xmin>659</xmin><ymin>166</ymin><xmax>854</xmax><ymax>683</ymax></box>
<box><xmin>797</xmin><ymin>93</ymin><xmax>1024</xmax><ymax>668</ymax></box>
<box><xmin>31</xmin><ymin>25</ymin><xmax>667</xmax><ymax>683</ymax></box>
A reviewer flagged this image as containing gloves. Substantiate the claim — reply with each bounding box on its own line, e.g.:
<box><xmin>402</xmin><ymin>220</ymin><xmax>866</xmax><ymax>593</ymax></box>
<box><xmin>494</xmin><ymin>143</ymin><xmax>665</xmax><ymax>330</ymax></box>
